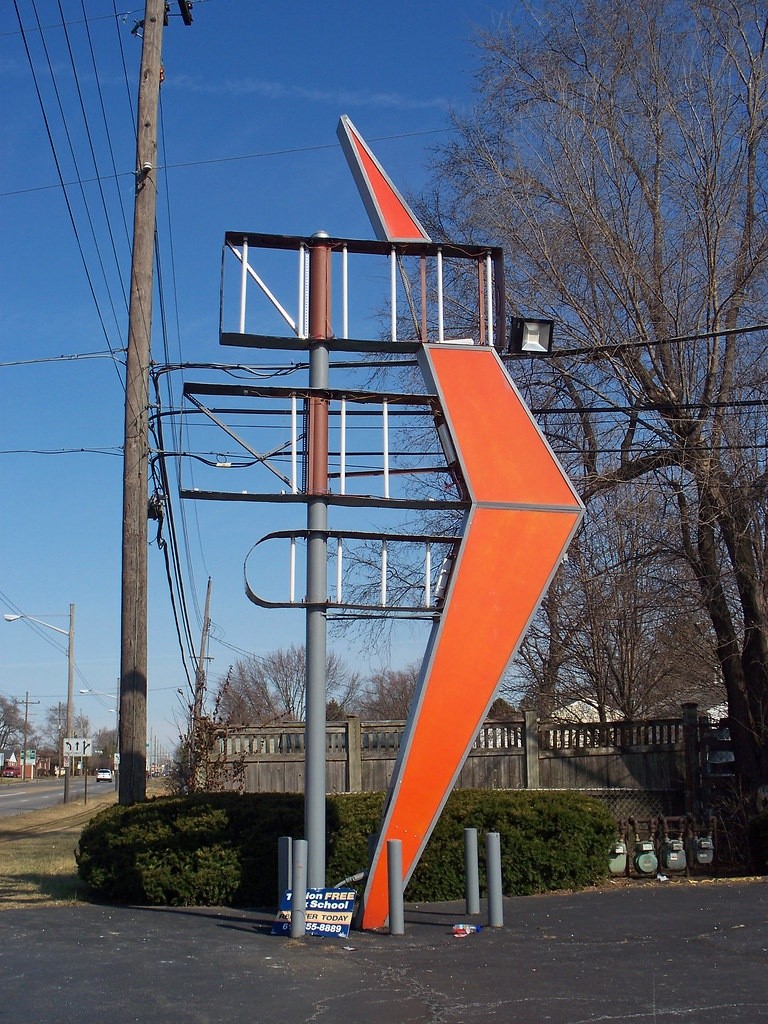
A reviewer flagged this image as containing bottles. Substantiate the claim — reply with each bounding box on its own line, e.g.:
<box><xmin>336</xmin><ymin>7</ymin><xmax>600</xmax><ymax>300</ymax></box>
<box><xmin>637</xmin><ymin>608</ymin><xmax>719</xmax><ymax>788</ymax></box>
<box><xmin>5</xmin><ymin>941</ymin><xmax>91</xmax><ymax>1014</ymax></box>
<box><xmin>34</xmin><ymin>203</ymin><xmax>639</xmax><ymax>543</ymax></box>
<box><xmin>453</xmin><ymin>924</ymin><xmax>481</xmax><ymax>933</ymax></box>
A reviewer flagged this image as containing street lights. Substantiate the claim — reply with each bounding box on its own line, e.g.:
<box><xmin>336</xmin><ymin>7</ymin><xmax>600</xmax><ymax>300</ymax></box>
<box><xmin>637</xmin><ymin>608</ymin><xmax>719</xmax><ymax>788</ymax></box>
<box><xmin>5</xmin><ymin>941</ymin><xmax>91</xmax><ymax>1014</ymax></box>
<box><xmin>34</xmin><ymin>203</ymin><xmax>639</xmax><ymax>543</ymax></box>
<box><xmin>4</xmin><ymin>604</ymin><xmax>75</xmax><ymax>804</ymax></box>
<box><xmin>79</xmin><ymin>677</ymin><xmax>120</xmax><ymax>791</ymax></box>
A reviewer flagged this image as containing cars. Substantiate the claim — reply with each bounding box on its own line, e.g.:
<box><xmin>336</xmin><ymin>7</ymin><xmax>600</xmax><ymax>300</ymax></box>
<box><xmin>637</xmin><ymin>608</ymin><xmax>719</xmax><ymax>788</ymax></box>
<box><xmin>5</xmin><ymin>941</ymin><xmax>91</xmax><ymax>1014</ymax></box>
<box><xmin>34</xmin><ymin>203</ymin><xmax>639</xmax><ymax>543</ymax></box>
<box><xmin>1</xmin><ymin>766</ymin><xmax>20</xmax><ymax>777</ymax></box>
<box><xmin>97</xmin><ymin>769</ymin><xmax>112</xmax><ymax>783</ymax></box>
